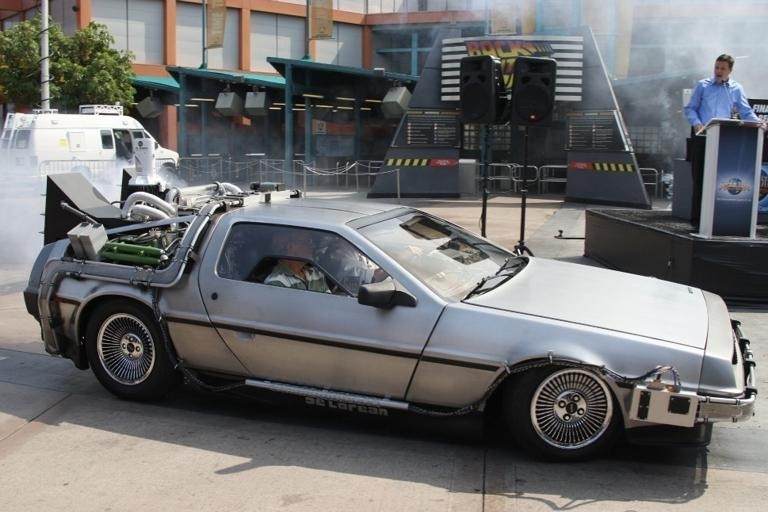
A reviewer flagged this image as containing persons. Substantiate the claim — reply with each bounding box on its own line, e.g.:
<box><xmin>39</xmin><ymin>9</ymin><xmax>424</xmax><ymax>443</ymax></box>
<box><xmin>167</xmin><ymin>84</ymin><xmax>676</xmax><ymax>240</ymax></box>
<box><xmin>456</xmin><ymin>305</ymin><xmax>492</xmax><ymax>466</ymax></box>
<box><xmin>686</xmin><ymin>53</ymin><xmax>762</xmax><ymax>133</ymax></box>
<box><xmin>325</xmin><ymin>232</ymin><xmax>421</xmax><ymax>284</ymax></box>
<box><xmin>263</xmin><ymin>229</ymin><xmax>333</xmax><ymax>294</ymax></box>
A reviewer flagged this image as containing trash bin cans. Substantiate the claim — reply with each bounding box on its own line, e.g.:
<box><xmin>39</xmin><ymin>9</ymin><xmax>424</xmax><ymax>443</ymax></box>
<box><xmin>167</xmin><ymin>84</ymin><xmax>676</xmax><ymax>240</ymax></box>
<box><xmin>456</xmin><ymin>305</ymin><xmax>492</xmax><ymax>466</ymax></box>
<box><xmin>458</xmin><ymin>159</ymin><xmax>479</xmax><ymax>199</ymax></box>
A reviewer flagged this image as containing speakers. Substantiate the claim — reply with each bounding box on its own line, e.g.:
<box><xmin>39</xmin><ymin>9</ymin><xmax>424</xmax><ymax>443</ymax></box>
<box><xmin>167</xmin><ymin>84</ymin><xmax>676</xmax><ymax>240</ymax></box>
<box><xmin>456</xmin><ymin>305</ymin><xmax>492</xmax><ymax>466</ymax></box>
<box><xmin>510</xmin><ymin>55</ymin><xmax>556</xmax><ymax>128</ymax></box>
<box><xmin>460</xmin><ymin>56</ymin><xmax>510</xmax><ymax>124</ymax></box>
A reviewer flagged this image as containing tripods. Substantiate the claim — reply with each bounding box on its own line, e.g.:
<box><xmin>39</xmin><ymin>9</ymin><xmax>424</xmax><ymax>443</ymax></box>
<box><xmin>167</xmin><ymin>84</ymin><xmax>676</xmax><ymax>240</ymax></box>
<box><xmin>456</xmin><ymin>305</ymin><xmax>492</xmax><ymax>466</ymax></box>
<box><xmin>512</xmin><ymin>133</ymin><xmax>539</xmax><ymax>257</ymax></box>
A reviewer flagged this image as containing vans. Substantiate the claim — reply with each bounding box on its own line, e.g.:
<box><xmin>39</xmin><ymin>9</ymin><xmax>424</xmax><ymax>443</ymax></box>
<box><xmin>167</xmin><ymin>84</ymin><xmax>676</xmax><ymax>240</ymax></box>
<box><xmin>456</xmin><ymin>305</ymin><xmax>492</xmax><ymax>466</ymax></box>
<box><xmin>0</xmin><ymin>101</ymin><xmax>180</xmax><ymax>191</ymax></box>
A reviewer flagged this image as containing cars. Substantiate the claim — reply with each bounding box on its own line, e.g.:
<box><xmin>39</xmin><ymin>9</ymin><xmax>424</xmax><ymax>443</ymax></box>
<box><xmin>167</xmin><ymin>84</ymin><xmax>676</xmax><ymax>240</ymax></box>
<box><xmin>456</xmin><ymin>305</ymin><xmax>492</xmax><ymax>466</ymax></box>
<box><xmin>23</xmin><ymin>169</ymin><xmax>758</xmax><ymax>464</ymax></box>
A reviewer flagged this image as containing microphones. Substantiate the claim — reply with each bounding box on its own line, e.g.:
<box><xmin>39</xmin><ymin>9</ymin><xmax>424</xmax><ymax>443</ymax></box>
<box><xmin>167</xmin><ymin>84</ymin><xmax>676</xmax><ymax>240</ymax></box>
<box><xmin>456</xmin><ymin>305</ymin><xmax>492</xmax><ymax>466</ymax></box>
<box><xmin>721</xmin><ymin>79</ymin><xmax>736</xmax><ymax>120</ymax></box>
